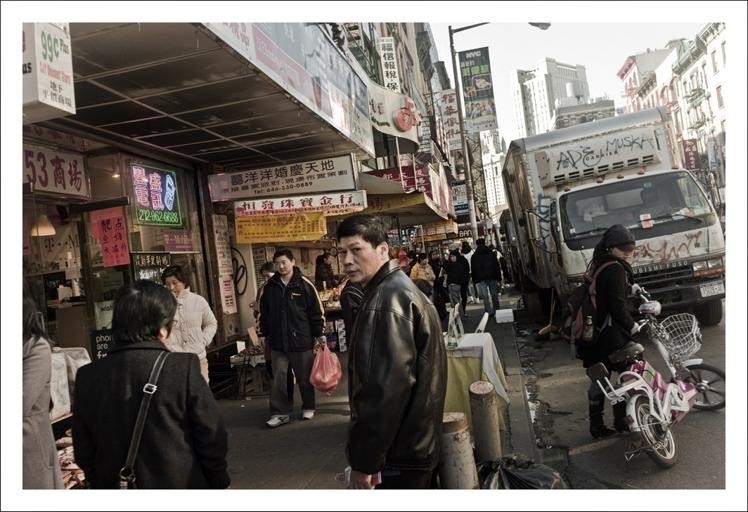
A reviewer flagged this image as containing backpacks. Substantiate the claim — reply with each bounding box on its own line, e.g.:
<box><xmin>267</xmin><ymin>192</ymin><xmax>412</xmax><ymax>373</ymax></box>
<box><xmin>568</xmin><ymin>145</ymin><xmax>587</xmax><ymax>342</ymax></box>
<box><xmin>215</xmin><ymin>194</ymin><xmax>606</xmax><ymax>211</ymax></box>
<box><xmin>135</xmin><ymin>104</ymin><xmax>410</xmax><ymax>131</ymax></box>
<box><xmin>573</xmin><ymin>260</ymin><xmax>629</xmax><ymax>360</ymax></box>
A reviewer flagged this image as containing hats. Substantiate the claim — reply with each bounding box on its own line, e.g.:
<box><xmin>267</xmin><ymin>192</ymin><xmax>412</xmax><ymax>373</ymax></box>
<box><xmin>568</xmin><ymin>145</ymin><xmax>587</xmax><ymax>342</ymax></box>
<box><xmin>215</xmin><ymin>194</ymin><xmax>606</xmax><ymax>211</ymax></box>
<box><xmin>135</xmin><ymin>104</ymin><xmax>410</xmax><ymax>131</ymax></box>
<box><xmin>605</xmin><ymin>224</ymin><xmax>635</xmax><ymax>248</ymax></box>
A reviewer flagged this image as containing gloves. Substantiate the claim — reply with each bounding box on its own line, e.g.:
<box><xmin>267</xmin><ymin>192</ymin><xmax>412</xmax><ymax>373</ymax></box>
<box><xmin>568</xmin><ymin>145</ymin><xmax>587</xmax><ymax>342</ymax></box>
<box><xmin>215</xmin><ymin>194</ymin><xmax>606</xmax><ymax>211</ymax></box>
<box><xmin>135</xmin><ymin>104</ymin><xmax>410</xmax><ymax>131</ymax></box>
<box><xmin>631</xmin><ymin>322</ymin><xmax>641</xmax><ymax>334</ymax></box>
<box><xmin>631</xmin><ymin>284</ymin><xmax>642</xmax><ymax>295</ymax></box>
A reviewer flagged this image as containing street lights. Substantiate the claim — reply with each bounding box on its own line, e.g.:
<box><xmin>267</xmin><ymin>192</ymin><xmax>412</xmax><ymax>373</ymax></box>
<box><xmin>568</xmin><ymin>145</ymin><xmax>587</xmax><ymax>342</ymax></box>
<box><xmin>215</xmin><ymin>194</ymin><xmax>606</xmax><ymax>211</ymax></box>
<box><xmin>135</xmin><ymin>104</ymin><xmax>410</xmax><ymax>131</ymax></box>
<box><xmin>446</xmin><ymin>22</ymin><xmax>549</xmax><ymax>250</ymax></box>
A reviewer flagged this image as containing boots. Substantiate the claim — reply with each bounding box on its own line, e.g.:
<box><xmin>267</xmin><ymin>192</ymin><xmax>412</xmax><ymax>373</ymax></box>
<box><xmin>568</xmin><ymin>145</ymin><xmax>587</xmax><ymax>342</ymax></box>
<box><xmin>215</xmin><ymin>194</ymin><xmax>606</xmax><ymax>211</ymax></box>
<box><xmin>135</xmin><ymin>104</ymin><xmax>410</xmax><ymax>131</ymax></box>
<box><xmin>612</xmin><ymin>396</ymin><xmax>631</xmax><ymax>434</ymax></box>
<box><xmin>588</xmin><ymin>392</ymin><xmax>617</xmax><ymax>439</ymax></box>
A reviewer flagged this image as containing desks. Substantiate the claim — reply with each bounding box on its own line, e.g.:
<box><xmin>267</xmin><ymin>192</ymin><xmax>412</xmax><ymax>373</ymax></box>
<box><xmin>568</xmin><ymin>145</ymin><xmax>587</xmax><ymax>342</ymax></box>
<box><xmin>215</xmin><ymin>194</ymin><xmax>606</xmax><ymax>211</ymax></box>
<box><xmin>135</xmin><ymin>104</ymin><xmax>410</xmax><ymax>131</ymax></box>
<box><xmin>442</xmin><ymin>333</ymin><xmax>510</xmax><ymax>435</ymax></box>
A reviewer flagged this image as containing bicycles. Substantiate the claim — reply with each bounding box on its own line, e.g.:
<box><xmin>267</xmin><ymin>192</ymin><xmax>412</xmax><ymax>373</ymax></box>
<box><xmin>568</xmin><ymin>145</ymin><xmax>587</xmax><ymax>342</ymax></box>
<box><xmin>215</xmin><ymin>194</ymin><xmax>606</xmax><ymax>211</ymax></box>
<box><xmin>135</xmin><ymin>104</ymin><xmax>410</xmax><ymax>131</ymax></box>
<box><xmin>585</xmin><ymin>286</ymin><xmax>723</xmax><ymax>467</ymax></box>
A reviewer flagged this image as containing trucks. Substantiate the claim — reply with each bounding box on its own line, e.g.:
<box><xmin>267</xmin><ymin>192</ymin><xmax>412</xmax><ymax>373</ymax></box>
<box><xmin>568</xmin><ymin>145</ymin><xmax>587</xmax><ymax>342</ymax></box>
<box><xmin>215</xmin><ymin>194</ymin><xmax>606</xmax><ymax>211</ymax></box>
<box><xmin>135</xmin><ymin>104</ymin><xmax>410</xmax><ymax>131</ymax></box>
<box><xmin>499</xmin><ymin>103</ymin><xmax>723</xmax><ymax>326</ymax></box>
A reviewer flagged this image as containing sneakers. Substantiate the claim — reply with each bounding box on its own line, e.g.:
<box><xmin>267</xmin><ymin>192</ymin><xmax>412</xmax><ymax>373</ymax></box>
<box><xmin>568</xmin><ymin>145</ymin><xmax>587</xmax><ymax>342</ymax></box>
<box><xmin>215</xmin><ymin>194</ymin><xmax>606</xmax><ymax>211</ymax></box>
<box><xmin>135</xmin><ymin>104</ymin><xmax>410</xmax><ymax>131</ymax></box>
<box><xmin>302</xmin><ymin>410</ymin><xmax>315</xmax><ymax>420</ymax></box>
<box><xmin>265</xmin><ymin>415</ymin><xmax>289</xmax><ymax>428</ymax></box>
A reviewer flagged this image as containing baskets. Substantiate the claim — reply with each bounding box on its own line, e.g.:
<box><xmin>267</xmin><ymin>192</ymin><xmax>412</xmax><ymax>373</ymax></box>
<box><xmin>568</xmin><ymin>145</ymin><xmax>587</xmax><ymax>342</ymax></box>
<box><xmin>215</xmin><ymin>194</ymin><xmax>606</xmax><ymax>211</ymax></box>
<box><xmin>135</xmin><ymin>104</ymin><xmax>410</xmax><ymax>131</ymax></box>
<box><xmin>659</xmin><ymin>313</ymin><xmax>703</xmax><ymax>363</ymax></box>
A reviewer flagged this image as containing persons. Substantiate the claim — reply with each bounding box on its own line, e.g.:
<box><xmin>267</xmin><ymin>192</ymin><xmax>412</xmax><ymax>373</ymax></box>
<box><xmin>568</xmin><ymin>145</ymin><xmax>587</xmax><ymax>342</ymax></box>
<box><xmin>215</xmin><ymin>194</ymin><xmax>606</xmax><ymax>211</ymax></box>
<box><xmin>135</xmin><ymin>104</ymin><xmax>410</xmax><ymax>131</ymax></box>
<box><xmin>155</xmin><ymin>264</ymin><xmax>219</xmax><ymax>387</ymax></box>
<box><xmin>23</xmin><ymin>277</ymin><xmax>68</xmax><ymax>489</ymax></box>
<box><xmin>251</xmin><ymin>247</ymin><xmax>366</xmax><ymax>427</ymax></box>
<box><xmin>69</xmin><ymin>279</ymin><xmax>233</xmax><ymax>488</ymax></box>
<box><xmin>333</xmin><ymin>211</ymin><xmax>449</xmax><ymax>490</ymax></box>
<box><xmin>572</xmin><ymin>222</ymin><xmax>644</xmax><ymax>436</ymax></box>
<box><xmin>399</xmin><ymin>235</ymin><xmax>509</xmax><ymax>320</ymax></box>
